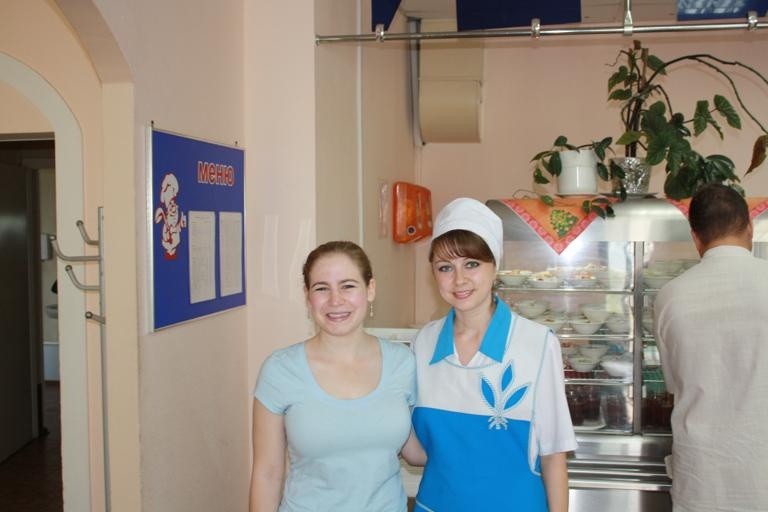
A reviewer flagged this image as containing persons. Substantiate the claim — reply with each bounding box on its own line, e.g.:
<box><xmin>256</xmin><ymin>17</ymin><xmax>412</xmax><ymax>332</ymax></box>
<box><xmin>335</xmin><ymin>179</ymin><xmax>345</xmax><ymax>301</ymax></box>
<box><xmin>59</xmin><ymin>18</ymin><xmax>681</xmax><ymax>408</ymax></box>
<box><xmin>652</xmin><ymin>180</ymin><xmax>766</xmax><ymax>512</ymax></box>
<box><xmin>411</xmin><ymin>197</ymin><xmax>580</xmax><ymax>512</ymax></box>
<box><xmin>248</xmin><ymin>240</ymin><xmax>428</xmax><ymax>512</ymax></box>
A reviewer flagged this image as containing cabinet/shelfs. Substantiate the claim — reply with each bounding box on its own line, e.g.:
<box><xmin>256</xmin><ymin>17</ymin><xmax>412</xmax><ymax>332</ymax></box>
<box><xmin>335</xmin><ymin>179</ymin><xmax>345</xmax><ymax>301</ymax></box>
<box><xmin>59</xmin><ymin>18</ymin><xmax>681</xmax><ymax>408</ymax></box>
<box><xmin>486</xmin><ymin>198</ymin><xmax>768</xmax><ymax>438</ymax></box>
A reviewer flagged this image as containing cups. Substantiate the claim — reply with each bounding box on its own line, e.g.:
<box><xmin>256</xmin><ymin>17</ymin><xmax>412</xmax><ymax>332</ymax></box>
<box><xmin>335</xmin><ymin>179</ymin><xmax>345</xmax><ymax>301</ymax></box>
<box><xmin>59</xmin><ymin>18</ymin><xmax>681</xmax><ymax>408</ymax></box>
<box><xmin>565</xmin><ymin>386</ymin><xmax>601</xmax><ymax>425</ymax></box>
<box><xmin>641</xmin><ymin>392</ymin><xmax>675</xmax><ymax>434</ymax></box>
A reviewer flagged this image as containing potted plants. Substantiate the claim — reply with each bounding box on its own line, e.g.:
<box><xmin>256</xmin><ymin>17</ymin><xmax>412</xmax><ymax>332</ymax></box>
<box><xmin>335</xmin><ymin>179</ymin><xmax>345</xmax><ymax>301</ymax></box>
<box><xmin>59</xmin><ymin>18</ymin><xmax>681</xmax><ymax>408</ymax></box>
<box><xmin>516</xmin><ymin>136</ymin><xmax>626</xmax><ymax>219</ymax></box>
<box><xmin>605</xmin><ymin>41</ymin><xmax>767</xmax><ymax>200</ymax></box>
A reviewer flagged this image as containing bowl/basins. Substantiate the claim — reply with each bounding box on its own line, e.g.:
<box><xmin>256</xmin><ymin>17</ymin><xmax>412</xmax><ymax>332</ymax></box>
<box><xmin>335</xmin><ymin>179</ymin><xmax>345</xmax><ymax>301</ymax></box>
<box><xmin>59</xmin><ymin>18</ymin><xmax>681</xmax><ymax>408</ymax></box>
<box><xmin>496</xmin><ymin>266</ymin><xmax>674</xmax><ymax>378</ymax></box>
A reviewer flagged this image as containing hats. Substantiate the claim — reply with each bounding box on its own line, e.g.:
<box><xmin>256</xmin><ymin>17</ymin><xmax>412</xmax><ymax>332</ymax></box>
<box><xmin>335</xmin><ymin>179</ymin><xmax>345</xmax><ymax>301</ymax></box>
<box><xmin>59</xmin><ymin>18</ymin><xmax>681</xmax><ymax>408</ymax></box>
<box><xmin>428</xmin><ymin>197</ymin><xmax>504</xmax><ymax>269</ymax></box>
